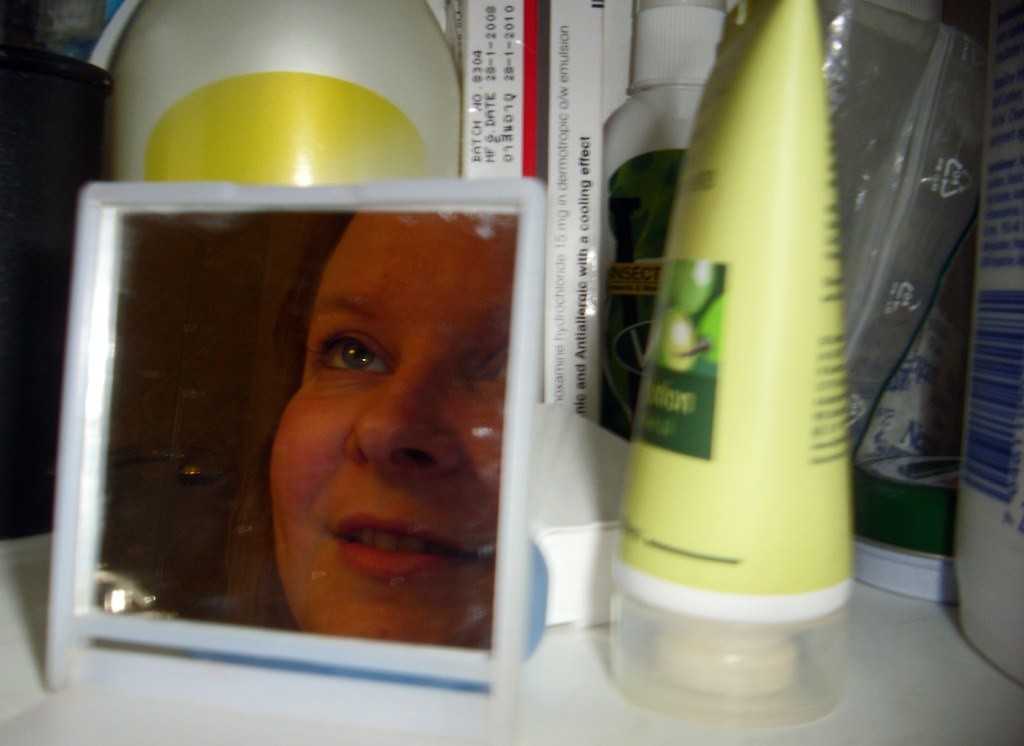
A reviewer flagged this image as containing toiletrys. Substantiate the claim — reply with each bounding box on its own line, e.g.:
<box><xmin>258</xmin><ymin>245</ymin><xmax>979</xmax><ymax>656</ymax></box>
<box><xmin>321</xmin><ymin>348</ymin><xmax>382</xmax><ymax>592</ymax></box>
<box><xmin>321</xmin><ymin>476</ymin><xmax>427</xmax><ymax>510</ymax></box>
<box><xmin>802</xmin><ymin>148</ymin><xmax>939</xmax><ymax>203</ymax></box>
<box><xmin>605</xmin><ymin>0</ymin><xmax>857</xmax><ymax>737</ymax></box>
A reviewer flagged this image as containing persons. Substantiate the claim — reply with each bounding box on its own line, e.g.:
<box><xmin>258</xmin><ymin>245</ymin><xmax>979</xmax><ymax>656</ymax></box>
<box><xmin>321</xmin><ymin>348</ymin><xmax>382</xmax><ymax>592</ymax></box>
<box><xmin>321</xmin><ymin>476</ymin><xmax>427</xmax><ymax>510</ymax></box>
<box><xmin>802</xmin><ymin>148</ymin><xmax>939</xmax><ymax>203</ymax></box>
<box><xmin>214</xmin><ymin>210</ymin><xmax>520</xmax><ymax>651</ymax></box>
